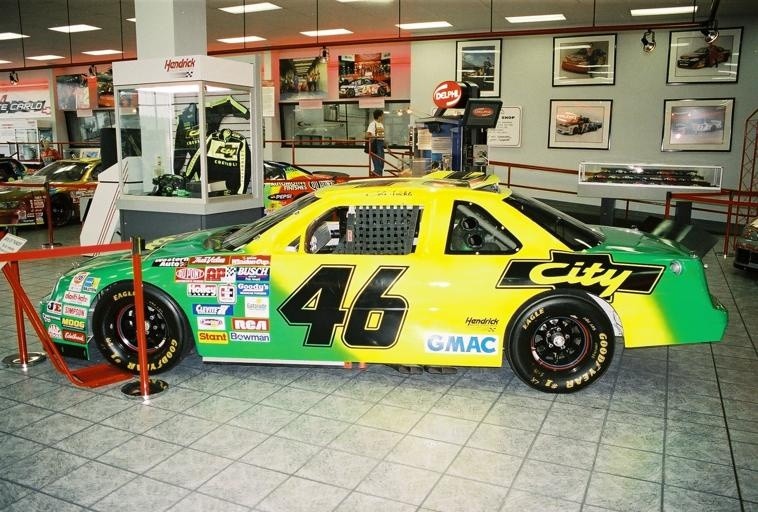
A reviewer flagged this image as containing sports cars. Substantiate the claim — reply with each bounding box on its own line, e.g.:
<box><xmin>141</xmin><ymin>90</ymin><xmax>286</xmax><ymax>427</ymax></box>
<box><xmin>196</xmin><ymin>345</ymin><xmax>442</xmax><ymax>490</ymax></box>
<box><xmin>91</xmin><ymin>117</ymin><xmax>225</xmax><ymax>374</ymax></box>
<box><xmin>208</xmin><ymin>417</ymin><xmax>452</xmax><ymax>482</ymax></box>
<box><xmin>562</xmin><ymin>46</ymin><xmax>608</xmax><ymax>76</ymax></box>
<box><xmin>677</xmin><ymin>45</ymin><xmax>727</xmax><ymax>68</ymax></box>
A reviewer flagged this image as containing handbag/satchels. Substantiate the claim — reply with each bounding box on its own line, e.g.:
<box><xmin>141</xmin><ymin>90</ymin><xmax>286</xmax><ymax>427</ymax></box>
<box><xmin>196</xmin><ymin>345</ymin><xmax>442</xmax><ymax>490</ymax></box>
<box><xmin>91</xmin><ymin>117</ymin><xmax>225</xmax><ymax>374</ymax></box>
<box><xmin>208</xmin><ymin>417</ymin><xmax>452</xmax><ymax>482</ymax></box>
<box><xmin>363</xmin><ymin>139</ymin><xmax>377</xmax><ymax>154</ymax></box>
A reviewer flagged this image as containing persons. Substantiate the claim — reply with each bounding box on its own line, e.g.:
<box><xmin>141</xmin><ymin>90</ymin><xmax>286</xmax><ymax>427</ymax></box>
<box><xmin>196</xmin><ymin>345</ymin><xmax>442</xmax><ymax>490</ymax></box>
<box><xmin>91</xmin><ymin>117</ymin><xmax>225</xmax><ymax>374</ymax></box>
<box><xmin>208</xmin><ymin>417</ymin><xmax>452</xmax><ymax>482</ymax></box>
<box><xmin>365</xmin><ymin>109</ymin><xmax>385</xmax><ymax>178</ymax></box>
<box><xmin>39</xmin><ymin>139</ymin><xmax>62</xmax><ymax>167</ymax></box>
<box><xmin>483</xmin><ymin>56</ymin><xmax>493</xmax><ymax>78</ymax></box>
<box><xmin>279</xmin><ymin>68</ymin><xmax>318</xmax><ymax>94</ymax></box>
<box><xmin>337</xmin><ymin>62</ymin><xmax>390</xmax><ymax>76</ymax></box>
<box><xmin>576</xmin><ymin>114</ymin><xmax>583</xmax><ymax>132</ymax></box>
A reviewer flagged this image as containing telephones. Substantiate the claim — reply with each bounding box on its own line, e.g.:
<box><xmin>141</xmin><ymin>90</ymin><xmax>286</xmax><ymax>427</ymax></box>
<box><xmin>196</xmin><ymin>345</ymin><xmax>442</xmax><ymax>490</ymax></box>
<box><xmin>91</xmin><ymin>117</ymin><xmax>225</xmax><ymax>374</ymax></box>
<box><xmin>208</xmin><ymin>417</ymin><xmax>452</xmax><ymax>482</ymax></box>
<box><xmin>152</xmin><ymin>174</ymin><xmax>184</xmax><ymax>196</ymax></box>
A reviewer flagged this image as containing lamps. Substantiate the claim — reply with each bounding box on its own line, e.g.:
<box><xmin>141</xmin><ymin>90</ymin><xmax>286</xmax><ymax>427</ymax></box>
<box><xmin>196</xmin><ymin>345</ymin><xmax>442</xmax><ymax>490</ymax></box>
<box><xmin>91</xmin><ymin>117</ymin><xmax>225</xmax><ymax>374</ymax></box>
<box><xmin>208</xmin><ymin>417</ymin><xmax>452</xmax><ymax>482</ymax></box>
<box><xmin>10</xmin><ymin>71</ymin><xmax>19</xmax><ymax>86</ymax></box>
<box><xmin>320</xmin><ymin>46</ymin><xmax>329</xmax><ymax>64</ymax></box>
<box><xmin>641</xmin><ymin>29</ymin><xmax>656</xmax><ymax>53</ymax></box>
<box><xmin>89</xmin><ymin>64</ymin><xmax>97</xmax><ymax>79</ymax></box>
<box><xmin>701</xmin><ymin>20</ymin><xmax>720</xmax><ymax>44</ymax></box>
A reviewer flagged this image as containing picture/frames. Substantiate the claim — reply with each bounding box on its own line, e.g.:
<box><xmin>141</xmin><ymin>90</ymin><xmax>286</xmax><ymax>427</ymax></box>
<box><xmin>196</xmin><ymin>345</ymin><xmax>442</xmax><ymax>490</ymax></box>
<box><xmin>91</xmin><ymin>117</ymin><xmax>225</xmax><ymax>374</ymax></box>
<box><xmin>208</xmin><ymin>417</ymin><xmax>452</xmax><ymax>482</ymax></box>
<box><xmin>552</xmin><ymin>33</ymin><xmax>617</xmax><ymax>87</ymax></box>
<box><xmin>548</xmin><ymin>99</ymin><xmax>613</xmax><ymax>150</ymax></box>
<box><xmin>666</xmin><ymin>26</ymin><xmax>744</xmax><ymax>84</ymax></box>
<box><xmin>659</xmin><ymin>98</ymin><xmax>735</xmax><ymax>152</ymax></box>
<box><xmin>456</xmin><ymin>38</ymin><xmax>502</xmax><ymax>98</ymax></box>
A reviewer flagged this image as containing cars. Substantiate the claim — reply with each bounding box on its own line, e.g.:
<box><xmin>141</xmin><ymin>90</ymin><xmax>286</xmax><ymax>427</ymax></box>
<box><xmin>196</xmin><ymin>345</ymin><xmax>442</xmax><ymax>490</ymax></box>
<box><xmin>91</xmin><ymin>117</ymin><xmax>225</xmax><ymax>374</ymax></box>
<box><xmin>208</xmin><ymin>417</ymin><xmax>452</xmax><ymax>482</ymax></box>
<box><xmin>697</xmin><ymin>120</ymin><xmax>724</xmax><ymax>132</ymax></box>
<box><xmin>39</xmin><ymin>175</ymin><xmax>729</xmax><ymax>394</ymax></box>
<box><xmin>0</xmin><ymin>154</ymin><xmax>102</xmax><ymax>227</ymax></box>
<box><xmin>339</xmin><ymin>78</ymin><xmax>388</xmax><ymax>97</ymax></box>
<box><xmin>557</xmin><ymin>117</ymin><xmax>602</xmax><ymax>135</ymax></box>
<box><xmin>263</xmin><ymin>160</ymin><xmax>347</xmax><ymax>211</ymax></box>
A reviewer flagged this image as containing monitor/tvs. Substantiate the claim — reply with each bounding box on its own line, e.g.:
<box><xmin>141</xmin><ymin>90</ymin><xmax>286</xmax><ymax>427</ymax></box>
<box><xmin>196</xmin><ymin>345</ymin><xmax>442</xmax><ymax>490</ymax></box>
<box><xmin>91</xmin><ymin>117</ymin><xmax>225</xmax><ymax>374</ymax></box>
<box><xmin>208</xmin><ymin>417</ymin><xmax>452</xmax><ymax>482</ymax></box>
<box><xmin>463</xmin><ymin>98</ymin><xmax>503</xmax><ymax>129</ymax></box>
